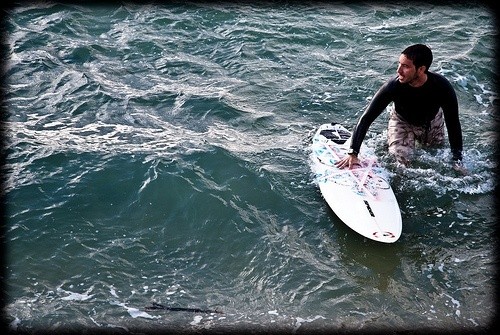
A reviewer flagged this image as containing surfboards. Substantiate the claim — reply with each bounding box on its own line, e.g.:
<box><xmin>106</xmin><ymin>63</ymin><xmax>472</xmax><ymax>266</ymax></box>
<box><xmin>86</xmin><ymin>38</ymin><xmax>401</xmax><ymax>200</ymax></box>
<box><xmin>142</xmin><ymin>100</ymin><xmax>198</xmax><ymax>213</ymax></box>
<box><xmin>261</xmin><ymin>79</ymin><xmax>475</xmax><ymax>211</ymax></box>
<box><xmin>313</xmin><ymin>122</ymin><xmax>403</xmax><ymax>243</ymax></box>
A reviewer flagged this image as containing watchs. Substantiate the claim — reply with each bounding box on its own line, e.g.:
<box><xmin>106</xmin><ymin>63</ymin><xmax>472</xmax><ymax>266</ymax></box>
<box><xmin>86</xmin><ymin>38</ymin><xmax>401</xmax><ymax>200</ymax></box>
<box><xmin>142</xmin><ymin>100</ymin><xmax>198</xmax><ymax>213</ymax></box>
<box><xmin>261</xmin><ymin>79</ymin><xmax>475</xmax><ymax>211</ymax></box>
<box><xmin>348</xmin><ymin>149</ymin><xmax>358</xmax><ymax>157</ymax></box>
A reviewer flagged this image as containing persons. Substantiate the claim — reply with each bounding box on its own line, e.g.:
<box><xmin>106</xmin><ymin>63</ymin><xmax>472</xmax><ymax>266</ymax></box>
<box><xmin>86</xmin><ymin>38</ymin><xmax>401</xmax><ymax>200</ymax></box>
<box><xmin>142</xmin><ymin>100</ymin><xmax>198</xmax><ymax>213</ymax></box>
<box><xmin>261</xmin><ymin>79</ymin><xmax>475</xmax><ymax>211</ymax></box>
<box><xmin>334</xmin><ymin>44</ymin><xmax>463</xmax><ymax>170</ymax></box>
